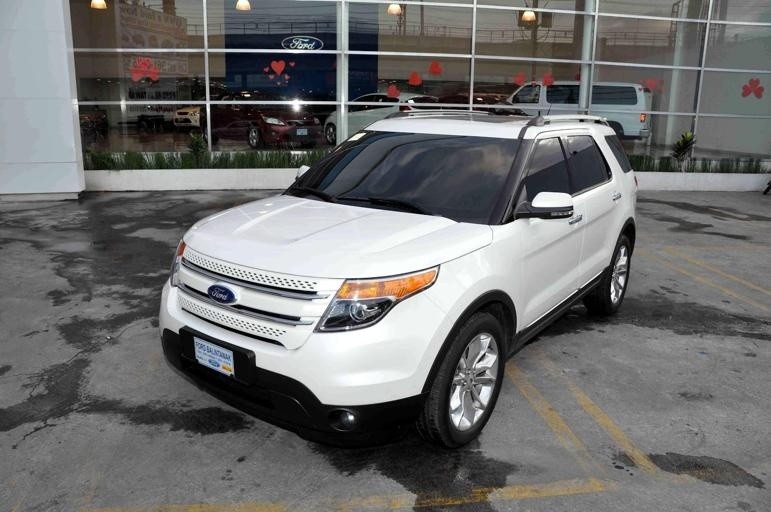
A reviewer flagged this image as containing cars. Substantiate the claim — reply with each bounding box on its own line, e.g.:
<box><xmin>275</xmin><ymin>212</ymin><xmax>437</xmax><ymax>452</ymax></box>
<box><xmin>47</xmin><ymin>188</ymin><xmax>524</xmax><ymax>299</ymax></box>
<box><xmin>436</xmin><ymin>94</ymin><xmax>528</xmax><ymax>117</ymax></box>
<box><xmin>79</xmin><ymin>97</ymin><xmax>108</xmax><ymax>135</ymax></box>
<box><xmin>324</xmin><ymin>92</ymin><xmax>440</xmax><ymax>147</ymax></box>
<box><xmin>200</xmin><ymin>92</ymin><xmax>323</xmax><ymax>149</ymax></box>
<box><xmin>294</xmin><ymin>94</ymin><xmax>349</xmax><ymax>126</ymax></box>
<box><xmin>173</xmin><ymin>95</ymin><xmax>220</xmax><ymax>134</ymax></box>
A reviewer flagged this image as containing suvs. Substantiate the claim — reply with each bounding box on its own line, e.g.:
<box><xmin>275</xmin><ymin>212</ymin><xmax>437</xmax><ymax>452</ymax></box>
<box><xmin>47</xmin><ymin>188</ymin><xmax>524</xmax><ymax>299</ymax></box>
<box><xmin>160</xmin><ymin>105</ymin><xmax>638</xmax><ymax>454</ymax></box>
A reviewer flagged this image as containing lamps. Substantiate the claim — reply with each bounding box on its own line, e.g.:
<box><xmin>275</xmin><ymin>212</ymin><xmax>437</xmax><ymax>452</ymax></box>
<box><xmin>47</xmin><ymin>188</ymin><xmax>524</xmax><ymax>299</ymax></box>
<box><xmin>522</xmin><ymin>9</ymin><xmax>537</xmax><ymax>21</ymax></box>
<box><xmin>89</xmin><ymin>0</ymin><xmax>107</xmax><ymax>9</ymax></box>
<box><xmin>235</xmin><ymin>0</ymin><xmax>252</xmax><ymax>12</ymax></box>
<box><xmin>386</xmin><ymin>4</ymin><xmax>403</xmax><ymax>15</ymax></box>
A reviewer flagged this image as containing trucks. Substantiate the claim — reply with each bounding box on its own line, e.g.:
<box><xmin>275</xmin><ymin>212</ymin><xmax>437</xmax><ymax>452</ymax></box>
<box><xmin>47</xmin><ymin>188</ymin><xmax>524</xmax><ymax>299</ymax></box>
<box><xmin>503</xmin><ymin>80</ymin><xmax>653</xmax><ymax>146</ymax></box>
